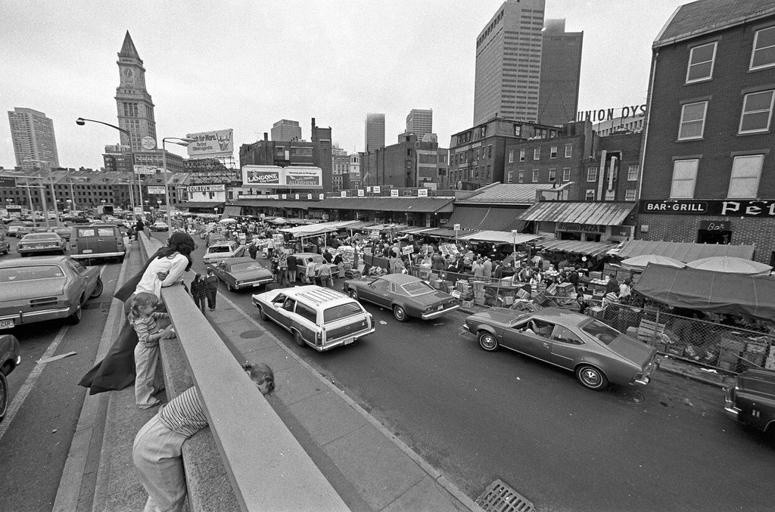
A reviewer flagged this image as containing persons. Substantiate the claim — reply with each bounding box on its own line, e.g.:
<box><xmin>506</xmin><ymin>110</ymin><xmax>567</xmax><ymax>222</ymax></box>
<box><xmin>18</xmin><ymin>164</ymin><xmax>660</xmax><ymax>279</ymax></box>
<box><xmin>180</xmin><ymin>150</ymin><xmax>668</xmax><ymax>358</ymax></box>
<box><xmin>131</xmin><ymin>216</ymin><xmax>144</xmax><ymax>241</ymax></box>
<box><xmin>530</xmin><ymin>320</ymin><xmax>554</xmax><ymax>338</ymax></box>
<box><xmin>203</xmin><ymin>268</ymin><xmax>219</xmax><ymax>312</ymax></box>
<box><xmin>130</xmin><ymin>359</ymin><xmax>276</xmax><ymax>512</ymax></box>
<box><xmin>190</xmin><ymin>272</ymin><xmax>208</xmax><ymax>318</ymax></box>
<box><xmin>180</xmin><ymin>279</ymin><xmax>193</xmax><ymax>297</ymax></box>
<box><xmin>122</xmin><ymin>230</ymin><xmax>195</xmax><ymax>320</ymax></box>
<box><xmin>145</xmin><ymin>209</ymin><xmax>233</xmax><ymax>236</ymax></box>
<box><xmin>233</xmin><ymin>213</ymin><xmax>632</xmax><ymax>314</ymax></box>
<box><xmin>127</xmin><ymin>291</ymin><xmax>174</xmax><ymax>411</ymax></box>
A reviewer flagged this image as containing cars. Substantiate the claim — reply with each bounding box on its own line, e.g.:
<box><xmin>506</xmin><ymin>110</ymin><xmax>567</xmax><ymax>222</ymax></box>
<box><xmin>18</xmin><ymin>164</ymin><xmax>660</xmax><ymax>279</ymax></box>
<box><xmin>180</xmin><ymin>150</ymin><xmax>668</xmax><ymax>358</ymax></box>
<box><xmin>718</xmin><ymin>366</ymin><xmax>775</xmax><ymax>445</ymax></box>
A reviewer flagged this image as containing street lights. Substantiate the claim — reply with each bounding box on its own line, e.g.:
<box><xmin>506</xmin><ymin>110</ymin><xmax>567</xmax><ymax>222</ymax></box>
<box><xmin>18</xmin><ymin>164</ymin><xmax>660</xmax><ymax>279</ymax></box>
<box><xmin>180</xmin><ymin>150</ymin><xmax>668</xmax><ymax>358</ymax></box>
<box><xmin>161</xmin><ymin>134</ymin><xmax>194</xmax><ymax>244</ymax></box>
<box><xmin>75</xmin><ymin>117</ymin><xmax>142</xmax><ymax>228</ymax></box>
